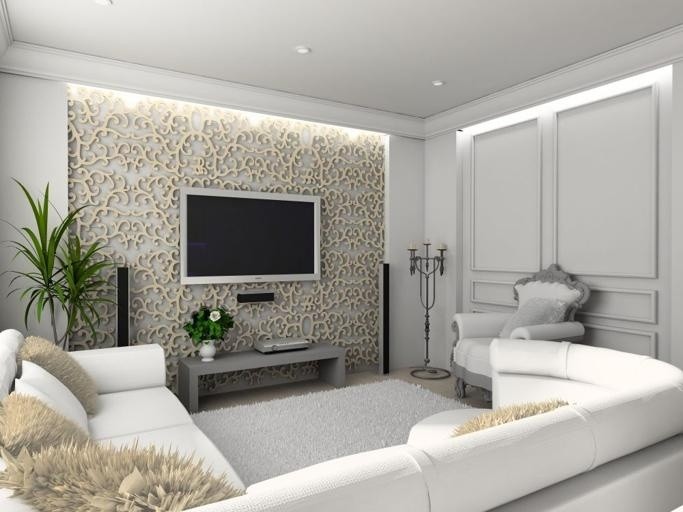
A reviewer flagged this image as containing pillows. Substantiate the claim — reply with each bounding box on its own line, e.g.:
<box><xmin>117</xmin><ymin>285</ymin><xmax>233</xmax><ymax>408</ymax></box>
<box><xmin>449</xmin><ymin>402</ymin><xmax>568</xmax><ymax>436</ymax></box>
<box><xmin>498</xmin><ymin>297</ymin><xmax>571</xmax><ymax>339</ymax></box>
<box><xmin>13</xmin><ymin>334</ymin><xmax>106</xmax><ymax>442</ymax></box>
<box><xmin>2</xmin><ymin>436</ymin><xmax>245</xmax><ymax>512</ymax></box>
<box><xmin>2</xmin><ymin>392</ymin><xmax>100</xmax><ymax>462</ymax></box>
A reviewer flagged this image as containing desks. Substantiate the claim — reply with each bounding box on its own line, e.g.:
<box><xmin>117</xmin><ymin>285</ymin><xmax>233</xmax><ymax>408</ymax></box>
<box><xmin>178</xmin><ymin>344</ymin><xmax>347</xmax><ymax>412</ymax></box>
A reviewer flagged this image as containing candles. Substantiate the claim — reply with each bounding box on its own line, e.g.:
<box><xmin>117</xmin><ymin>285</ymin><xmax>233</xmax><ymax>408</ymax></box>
<box><xmin>408</xmin><ymin>239</ymin><xmax>446</xmax><ymax>250</ymax></box>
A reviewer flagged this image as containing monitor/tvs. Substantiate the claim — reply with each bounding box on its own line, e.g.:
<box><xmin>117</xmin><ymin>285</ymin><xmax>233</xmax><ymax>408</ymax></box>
<box><xmin>179</xmin><ymin>187</ymin><xmax>322</xmax><ymax>286</ymax></box>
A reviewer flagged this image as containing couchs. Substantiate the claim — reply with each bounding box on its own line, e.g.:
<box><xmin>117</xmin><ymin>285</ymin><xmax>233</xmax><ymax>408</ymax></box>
<box><xmin>0</xmin><ymin>326</ymin><xmax>682</xmax><ymax>512</ymax></box>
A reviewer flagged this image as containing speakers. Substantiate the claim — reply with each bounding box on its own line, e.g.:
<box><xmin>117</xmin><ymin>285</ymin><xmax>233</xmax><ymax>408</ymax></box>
<box><xmin>379</xmin><ymin>263</ymin><xmax>389</xmax><ymax>374</ymax></box>
<box><xmin>237</xmin><ymin>292</ymin><xmax>274</xmax><ymax>303</ymax></box>
<box><xmin>116</xmin><ymin>266</ymin><xmax>131</xmax><ymax>347</ymax></box>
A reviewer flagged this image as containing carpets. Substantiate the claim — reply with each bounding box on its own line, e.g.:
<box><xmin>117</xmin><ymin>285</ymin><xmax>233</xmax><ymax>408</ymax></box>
<box><xmin>188</xmin><ymin>376</ymin><xmax>473</xmax><ymax>486</ymax></box>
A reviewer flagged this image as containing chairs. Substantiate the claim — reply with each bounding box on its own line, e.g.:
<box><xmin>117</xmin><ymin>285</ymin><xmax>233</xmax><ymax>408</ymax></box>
<box><xmin>449</xmin><ymin>261</ymin><xmax>591</xmax><ymax>401</ymax></box>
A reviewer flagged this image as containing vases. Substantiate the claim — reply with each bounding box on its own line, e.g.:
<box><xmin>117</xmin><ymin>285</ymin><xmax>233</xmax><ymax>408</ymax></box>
<box><xmin>198</xmin><ymin>339</ymin><xmax>217</xmax><ymax>362</ymax></box>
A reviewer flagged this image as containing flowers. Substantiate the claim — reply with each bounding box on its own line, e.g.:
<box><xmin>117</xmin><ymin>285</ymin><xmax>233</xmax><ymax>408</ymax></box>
<box><xmin>183</xmin><ymin>304</ymin><xmax>234</xmax><ymax>345</ymax></box>
